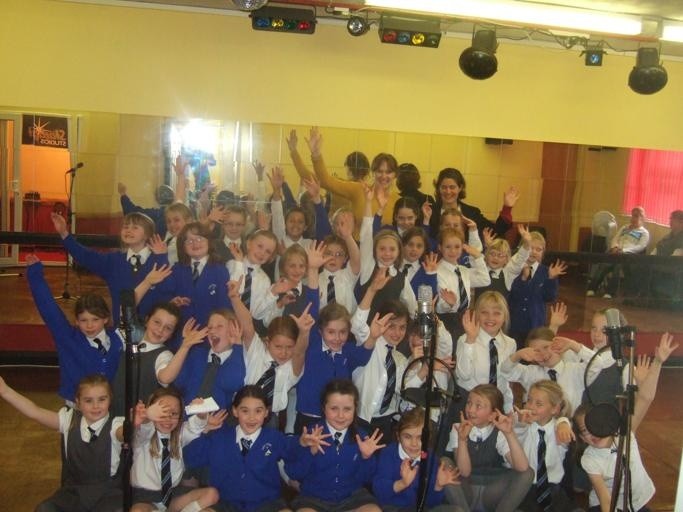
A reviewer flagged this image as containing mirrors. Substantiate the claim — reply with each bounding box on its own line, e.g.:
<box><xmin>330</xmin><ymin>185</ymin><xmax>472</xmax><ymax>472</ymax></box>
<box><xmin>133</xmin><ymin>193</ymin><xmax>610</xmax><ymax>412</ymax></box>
<box><xmin>0</xmin><ymin>108</ymin><xmax>682</xmax><ymax>361</ymax></box>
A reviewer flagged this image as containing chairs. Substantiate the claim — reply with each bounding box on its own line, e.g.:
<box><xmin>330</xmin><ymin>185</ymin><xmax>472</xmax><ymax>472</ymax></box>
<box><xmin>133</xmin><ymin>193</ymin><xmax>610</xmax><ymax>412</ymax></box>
<box><xmin>644</xmin><ymin>251</ymin><xmax>683</xmax><ymax>311</ymax></box>
<box><xmin>602</xmin><ymin>244</ymin><xmax>646</xmax><ymax>301</ymax></box>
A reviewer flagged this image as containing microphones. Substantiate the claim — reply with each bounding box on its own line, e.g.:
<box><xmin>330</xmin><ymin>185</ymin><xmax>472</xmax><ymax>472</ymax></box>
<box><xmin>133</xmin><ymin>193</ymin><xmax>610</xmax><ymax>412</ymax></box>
<box><xmin>602</xmin><ymin>308</ymin><xmax>624</xmax><ymax>373</ymax></box>
<box><xmin>120</xmin><ymin>288</ymin><xmax>142</xmax><ymax>359</ymax></box>
<box><xmin>414</xmin><ymin>283</ymin><xmax>436</xmax><ymax>348</ymax></box>
<box><xmin>65</xmin><ymin>162</ymin><xmax>84</xmax><ymax>175</ymax></box>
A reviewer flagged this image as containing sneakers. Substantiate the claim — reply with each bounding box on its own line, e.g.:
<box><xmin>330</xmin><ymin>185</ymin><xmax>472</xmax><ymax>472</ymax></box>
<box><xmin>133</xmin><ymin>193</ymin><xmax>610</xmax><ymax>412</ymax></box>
<box><xmin>586</xmin><ymin>279</ymin><xmax>597</xmax><ymax>299</ymax></box>
<box><xmin>603</xmin><ymin>284</ymin><xmax>615</xmax><ymax>300</ymax></box>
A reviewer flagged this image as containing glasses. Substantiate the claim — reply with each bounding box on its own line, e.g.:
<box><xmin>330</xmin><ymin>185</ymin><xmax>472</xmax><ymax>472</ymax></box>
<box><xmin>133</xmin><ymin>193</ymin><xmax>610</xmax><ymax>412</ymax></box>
<box><xmin>181</xmin><ymin>234</ymin><xmax>206</xmax><ymax>244</ymax></box>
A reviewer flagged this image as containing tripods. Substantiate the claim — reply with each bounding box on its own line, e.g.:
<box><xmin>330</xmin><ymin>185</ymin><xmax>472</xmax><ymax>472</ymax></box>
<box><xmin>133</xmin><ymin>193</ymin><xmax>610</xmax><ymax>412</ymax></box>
<box><xmin>51</xmin><ymin>175</ymin><xmax>83</xmax><ymax>302</ymax></box>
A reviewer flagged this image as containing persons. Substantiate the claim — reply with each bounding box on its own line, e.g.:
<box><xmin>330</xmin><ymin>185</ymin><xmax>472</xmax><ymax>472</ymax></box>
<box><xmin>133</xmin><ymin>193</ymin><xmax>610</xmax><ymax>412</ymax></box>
<box><xmin>2</xmin><ymin>126</ymin><xmax>683</xmax><ymax>512</ymax></box>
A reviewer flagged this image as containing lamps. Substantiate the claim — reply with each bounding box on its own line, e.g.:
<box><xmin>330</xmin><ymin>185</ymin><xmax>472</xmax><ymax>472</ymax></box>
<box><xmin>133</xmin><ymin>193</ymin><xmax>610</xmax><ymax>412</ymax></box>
<box><xmin>346</xmin><ymin>1</ymin><xmax>372</xmax><ymax>37</ymax></box>
<box><xmin>376</xmin><ymin>5</ymin><xmax>444</xmax><ymax>48</ymax></box>
<box><xmin>626</xmin><ymin>46</ymin><xmax>667</xmax><ymax>95</ymax></box>
<box><xmin>578</xmin><ymin>35</ymin><xmax>609</xmax><ymax>69</ymax></box>
<box><xmin>235</xmin><ymin>0</ymin><xmax>269</xmax><ymax>12</ymax></box>
<box><xmin>247</xmin><ymin>1</ymin><xmax>319</xmax><ymax>38</ymax></box>
<box><xmin>365</xmin><ymin>1</ymin><xmax>662</xmax><ymax>47</ymax></box>
<box><xmin>457</xmin><ymin>20</ymin><xmax>502</xmax><ymax>81</ymax></box>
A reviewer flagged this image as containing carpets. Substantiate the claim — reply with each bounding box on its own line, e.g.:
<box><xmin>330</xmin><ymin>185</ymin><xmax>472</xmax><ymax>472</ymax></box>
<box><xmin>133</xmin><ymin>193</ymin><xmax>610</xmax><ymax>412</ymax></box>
<box><xmin>1</xmin><ymin>322</ymin><xmax>61</xmax><ymax>352</ymax></box>
<box><xmin>555</xmin><ymin>323</ymin><xmax>681</xmax><ymax>360</ymax></box>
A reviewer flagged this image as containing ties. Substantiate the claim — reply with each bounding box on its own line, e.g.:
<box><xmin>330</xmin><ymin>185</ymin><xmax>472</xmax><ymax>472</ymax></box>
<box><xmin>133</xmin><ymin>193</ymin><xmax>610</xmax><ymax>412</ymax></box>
<box><xmin>333</xmin><ymin>430</ymin><xmax>342</xmax><ymax>453</ymax></box>
<box><xmin>487</xmin><ymin>337</ymin><xmax>499</xmax><ymax>389</ymax></box>
<box><xmin>547</xmin><ymin>369</ymin><xmax>557</xmax><ymax>384</ymax></box>
<box><xmin>535</xmin><ymin>429</ymin><xmax>554</xmax><ymax>511</ymax></box>
<box><xmin>159</xmin><ymin>436</ymin><xmax>175</xmax><ymax>509</ymax></box>
<box><xmin>527</xmin><ymin>264</ymin><xmax>534</xmax><ymax>281</ymax></box>
<box><xmin>453</xmin><ymin>265</ymin><xmax>469</xmax><ymax>316</ymax></box>
<box><xmin>379</xmin><ymin>343</ymin><xmax>398</xmax><ymax>416</ymax></box>
<box><xmin>239</xmin><ymin>437</ymin><xmax>253</xmax><ymax>456</ymax></box>
<box><xmin>402</xmin><ymin>262</ymin><xmax>414</xmax><ymax>277</ymax></box>
<box><xmin>129</xmin><ymin>235</ymin><xmax>339</xmax><ymax>313</ymax></box>
<box><xmin>87</xmin><ymin>426</ymin><xmax>97</xmax><ymax>444</ymax></box>
<box><xmin>93</xmin><ymin>336</ymin><xmax>108</xmax><ymax>355</ymax></box>
<box><xmin>199</xmin><ymin>353</ymin><xmax>220</xmax><ymax>400</ymax></box>
<box><xmin>255</xmin><ymin>358</ymin><xmax>281</xmax><ymax>415</ymax></box>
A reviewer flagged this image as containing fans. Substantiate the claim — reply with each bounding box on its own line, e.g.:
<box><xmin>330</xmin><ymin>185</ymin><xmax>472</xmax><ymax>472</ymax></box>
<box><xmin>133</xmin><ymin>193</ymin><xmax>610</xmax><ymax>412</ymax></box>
<box><xmin>587</xmin><ymin>209</ymin><xmax>616</xmax><ymax>290</ymax></box>
